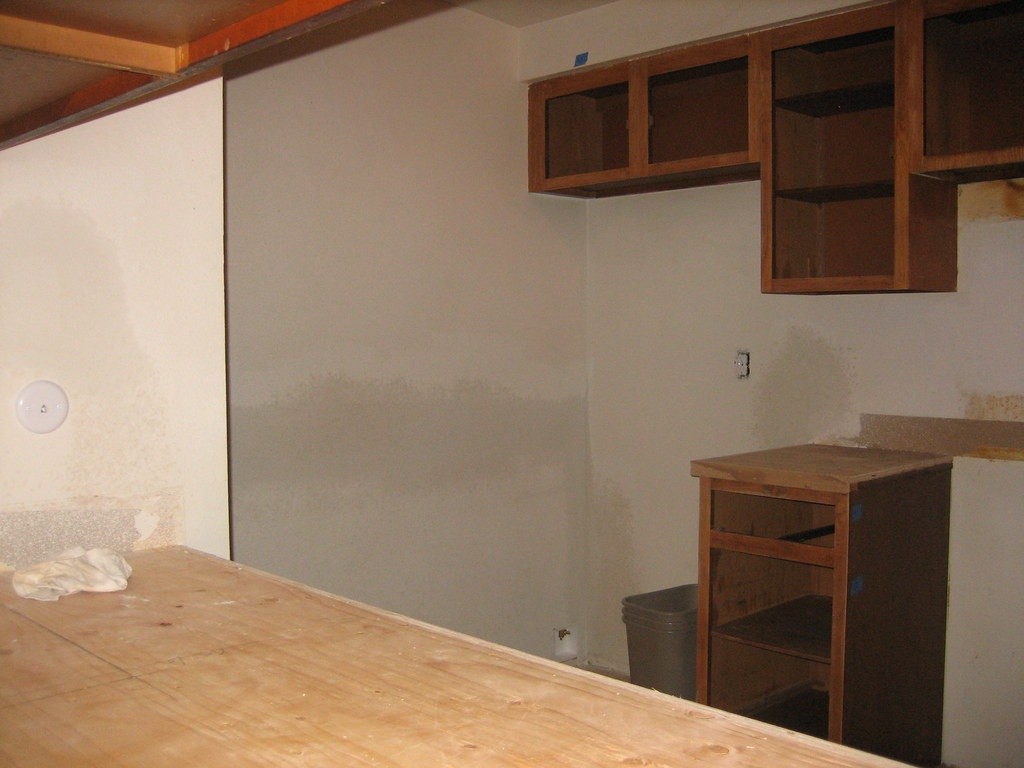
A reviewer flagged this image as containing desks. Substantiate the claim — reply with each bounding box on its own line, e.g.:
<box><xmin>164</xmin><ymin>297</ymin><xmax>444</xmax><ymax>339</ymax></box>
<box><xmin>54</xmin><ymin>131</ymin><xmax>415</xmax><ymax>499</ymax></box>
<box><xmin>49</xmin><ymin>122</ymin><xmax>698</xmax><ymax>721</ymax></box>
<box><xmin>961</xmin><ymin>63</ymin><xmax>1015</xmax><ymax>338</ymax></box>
<box><xmin>0</xmin><ymin>544</ymin><xmax>930</xmax><ymax>768</ymax></box>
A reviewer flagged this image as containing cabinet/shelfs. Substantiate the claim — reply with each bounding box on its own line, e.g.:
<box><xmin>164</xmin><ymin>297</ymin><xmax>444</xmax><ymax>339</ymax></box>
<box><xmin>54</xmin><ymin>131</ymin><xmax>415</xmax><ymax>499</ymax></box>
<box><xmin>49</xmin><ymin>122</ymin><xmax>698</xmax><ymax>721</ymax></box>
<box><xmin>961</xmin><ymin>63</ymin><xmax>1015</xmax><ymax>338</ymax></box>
<box><xmin>691</xmin><ymin>442</ymin><xmax>953</xmax><ymax>768</ymax></box>
<box><xmin>527</xmin><ymin>0</ymin><xmax>1024</xmax><ymax>296</ymax></box>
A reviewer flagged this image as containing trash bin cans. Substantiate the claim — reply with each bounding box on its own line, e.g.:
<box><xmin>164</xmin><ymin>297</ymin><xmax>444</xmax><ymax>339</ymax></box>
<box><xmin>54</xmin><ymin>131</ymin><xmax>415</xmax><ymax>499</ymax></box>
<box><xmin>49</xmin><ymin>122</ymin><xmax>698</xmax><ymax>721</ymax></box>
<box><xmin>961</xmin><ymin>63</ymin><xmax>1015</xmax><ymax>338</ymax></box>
<box><xmin>621</xmin><ymin>583</ymin><xmax>697</xmax><ymax>703</ymax></box>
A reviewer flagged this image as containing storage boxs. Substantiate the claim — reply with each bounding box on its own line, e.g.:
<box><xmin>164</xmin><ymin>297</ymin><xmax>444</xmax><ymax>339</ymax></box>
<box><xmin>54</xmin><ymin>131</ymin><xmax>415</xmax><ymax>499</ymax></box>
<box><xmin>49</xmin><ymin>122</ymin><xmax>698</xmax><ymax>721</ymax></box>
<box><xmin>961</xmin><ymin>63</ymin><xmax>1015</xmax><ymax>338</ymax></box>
<box><xmin>620</xmin><ymin>583</ymin><xmax>699</xmax><ymax>701</ymax></box>
